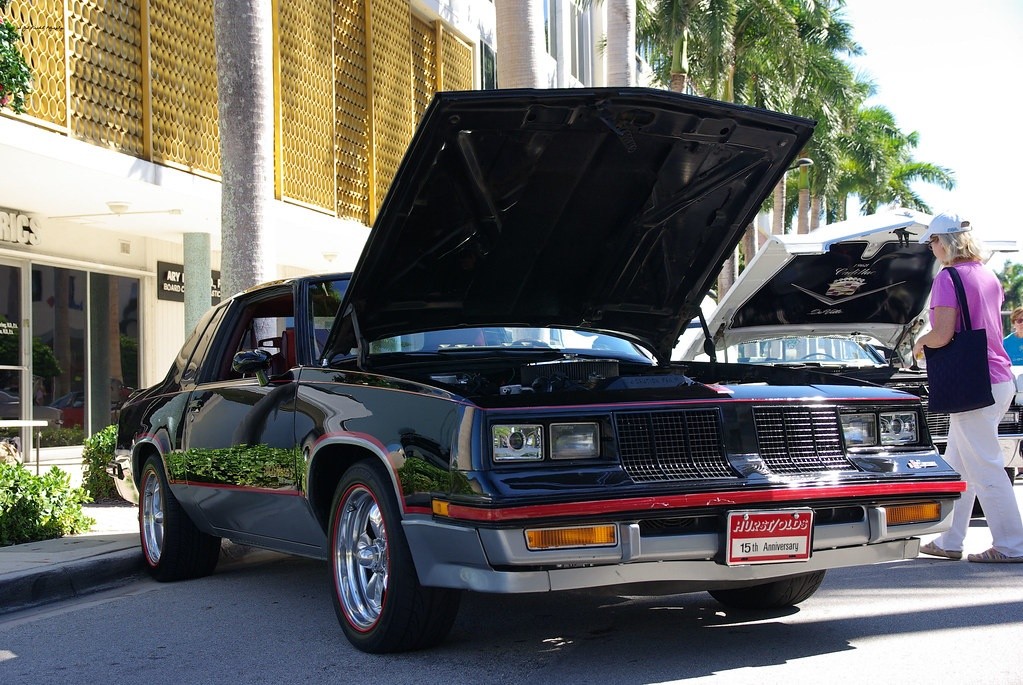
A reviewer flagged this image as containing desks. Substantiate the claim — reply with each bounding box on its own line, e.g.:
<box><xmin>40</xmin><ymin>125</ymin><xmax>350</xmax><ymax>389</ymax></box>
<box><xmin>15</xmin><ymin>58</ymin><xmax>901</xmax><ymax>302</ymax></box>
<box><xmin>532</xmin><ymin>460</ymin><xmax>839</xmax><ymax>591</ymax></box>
<box><xmin>0</xmin><ymin>420</ymin><xmax>48</xmax><ymax>475</ymax></box>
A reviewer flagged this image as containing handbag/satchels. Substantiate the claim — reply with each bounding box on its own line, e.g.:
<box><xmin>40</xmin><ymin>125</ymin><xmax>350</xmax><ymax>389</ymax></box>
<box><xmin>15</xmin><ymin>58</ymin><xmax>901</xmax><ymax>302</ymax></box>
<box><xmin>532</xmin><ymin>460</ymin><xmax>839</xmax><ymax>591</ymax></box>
<box><xmin>923</xmin><ymin>267</ymin><xmax>996</xmax><ymax>413</ymax></box>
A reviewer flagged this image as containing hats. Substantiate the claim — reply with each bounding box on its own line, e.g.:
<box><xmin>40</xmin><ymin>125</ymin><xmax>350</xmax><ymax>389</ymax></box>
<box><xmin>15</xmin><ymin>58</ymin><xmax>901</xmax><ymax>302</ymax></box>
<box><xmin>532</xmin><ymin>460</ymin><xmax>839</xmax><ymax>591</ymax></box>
<box><xmin>919</xmin><ymin>208</ymin><xmax>973</xmax><ymax>244</ymax></box>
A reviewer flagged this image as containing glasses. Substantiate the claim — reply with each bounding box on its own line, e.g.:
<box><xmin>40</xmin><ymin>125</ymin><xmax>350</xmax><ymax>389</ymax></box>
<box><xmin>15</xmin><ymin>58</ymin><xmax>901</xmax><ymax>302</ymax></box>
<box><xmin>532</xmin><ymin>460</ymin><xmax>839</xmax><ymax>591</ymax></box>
<box><xmin>1015</xmin><ymin>319</ymin><xmax>1023</xmax><ymax>323</ymax></box>
<box><xmin>928</xmin><ymin>240</ymin><xmax>934</xmax><ymax>250</ymax></box>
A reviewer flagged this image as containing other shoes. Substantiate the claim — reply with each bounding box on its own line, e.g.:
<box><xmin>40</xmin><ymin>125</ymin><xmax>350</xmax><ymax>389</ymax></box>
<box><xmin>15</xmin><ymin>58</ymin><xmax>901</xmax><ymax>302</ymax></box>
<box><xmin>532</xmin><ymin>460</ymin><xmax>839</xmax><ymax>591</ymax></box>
<box><xmin>920</xmin><ymin>541</ymin><xmax>962</xmax><ymax>560</ymax></box>
<box><xmin>968</xmin><ymin>548</ymin><xmax>1023</xmax><ymax>562</ymax></box>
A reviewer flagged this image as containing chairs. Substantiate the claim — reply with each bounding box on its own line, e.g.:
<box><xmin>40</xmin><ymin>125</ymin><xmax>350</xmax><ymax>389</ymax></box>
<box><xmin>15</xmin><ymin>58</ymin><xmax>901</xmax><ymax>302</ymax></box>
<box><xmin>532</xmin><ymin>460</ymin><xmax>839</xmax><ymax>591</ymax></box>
<box><xmin>282</xmin><ymin>328</ymin><xmax>351</xmax><ymax>365</ymax></box>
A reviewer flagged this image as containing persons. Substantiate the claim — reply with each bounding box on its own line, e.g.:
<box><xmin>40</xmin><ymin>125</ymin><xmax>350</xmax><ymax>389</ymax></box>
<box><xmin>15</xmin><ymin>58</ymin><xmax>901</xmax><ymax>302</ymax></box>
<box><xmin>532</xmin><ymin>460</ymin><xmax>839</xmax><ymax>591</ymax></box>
<box><xmin>910</xmin><ymin>208</ymin><xmax>1023</xmax><ymax>565</ymax></box>
<box><xmin>1002</xmin><ymin>307</ymin><xmax>1023</xmax><ymax>366</ymax></box>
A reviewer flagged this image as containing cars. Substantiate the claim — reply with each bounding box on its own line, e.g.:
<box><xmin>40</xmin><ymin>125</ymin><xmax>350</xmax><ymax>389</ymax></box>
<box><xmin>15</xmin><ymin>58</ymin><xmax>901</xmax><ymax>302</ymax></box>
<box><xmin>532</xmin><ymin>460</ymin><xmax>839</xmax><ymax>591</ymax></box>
<box><xmin>0</xmin><ymin>366</ymin><xmax>64</xmax><ymax>430</ymax></box>
<box><xmin>106</xmin><ymin>88</ymin><xmax>965</xmax><ymax>653</ymax></box>
<box><xmin>670</xmin><ymin>210</ymin><xmax>1023</xmax><ymax>513</ymax></box>
<box><xmin>48</xmin><ymin>391</ymin><xmax>84</xmax><ymax>429</ymax></box>
